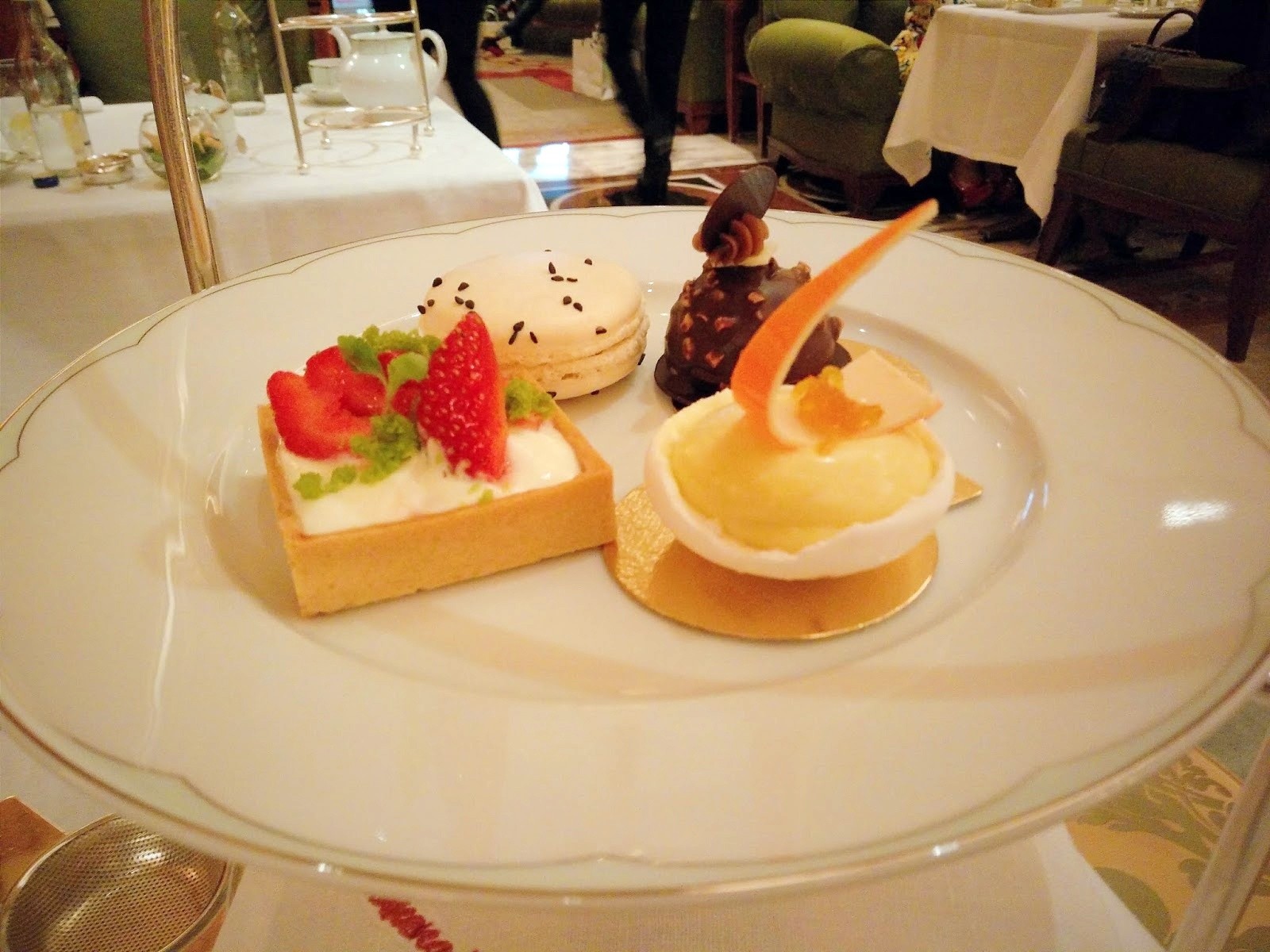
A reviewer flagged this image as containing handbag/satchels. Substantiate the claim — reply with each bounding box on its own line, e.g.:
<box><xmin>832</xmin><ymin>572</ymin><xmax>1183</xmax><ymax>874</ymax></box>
<box><xmin>1086</xmin><ymin>6</ymin><xmax>1255</xmax><ymax>158</ymax></box>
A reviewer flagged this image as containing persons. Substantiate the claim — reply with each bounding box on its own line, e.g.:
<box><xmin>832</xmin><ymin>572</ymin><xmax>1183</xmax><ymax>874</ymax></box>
<box><xmin>1057</xmin><ymin>0</ymin><xmax>1270</xmax><ymax>270</ymax></box>
<box><xmin>887</xmin><ymin>0</ymin><xmax>1019</xmax><ymax>209</ymax></box>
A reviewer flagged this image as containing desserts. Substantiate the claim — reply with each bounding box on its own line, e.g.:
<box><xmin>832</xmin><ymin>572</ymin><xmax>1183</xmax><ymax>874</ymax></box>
<box><xmin>222</xmin><ymin>153</ymin><xmax>967</xmax><ymax>658</ymax></box>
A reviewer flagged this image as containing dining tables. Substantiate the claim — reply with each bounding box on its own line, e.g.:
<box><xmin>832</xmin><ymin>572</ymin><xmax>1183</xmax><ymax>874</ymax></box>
<box><xmin>877</xmin><ymin>1</ymin><xmax>1200</xmax><ymax>249</ymax></box>
<box><xmin>1</xmin><ymin>90</ymin><xmax>539</xmax><ymax>417</ymax></box>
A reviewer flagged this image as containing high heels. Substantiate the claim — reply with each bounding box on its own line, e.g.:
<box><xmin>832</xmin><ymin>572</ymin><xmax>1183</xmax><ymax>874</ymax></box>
<box><xmin>981</xmin><ymin>165</ymin><xmax>1018</xmax><ymax>214</ymax></box>
<box><xmin>946</xmin><ymin>167</ymin><xmax>1000</xmax><ymax>221</ymax></box>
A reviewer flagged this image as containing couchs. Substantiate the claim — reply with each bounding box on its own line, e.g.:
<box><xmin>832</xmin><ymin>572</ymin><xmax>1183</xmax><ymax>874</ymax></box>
<box><xmin>748</xmin><ymin>0</ymin><xmax>962</xmax><ymax>220</ymax></box>
<box><xmin>634</xmin><ymin>1</ymin><xmax>722</xmax><ymax>132</ymax></box>
<box><xmin>522</xmin><ymin>0</ymin><xmax>605</xmax><ymax>47</ymax></box>
<box><xmin>1027</xmin><ymin>46</ymin><xmax>1270</xmax><ymax>361</ymax></box>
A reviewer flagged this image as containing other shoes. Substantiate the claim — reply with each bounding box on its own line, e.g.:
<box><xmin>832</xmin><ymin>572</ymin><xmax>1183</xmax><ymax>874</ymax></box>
<box><xmin>1080</xmin><ymin>233</ymin><xmax>1148</xmax><ymax>278</ymax></box>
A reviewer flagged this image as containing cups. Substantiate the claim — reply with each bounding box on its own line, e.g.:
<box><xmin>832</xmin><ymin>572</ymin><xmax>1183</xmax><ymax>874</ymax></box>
<box><xmin>308</xmin><ymin>57</ymin><xmax>340</xmax><ymax>90</ymax></box>
<box><xmin>0</xmin><ymin>107</ymin><xmax>40</xmax><ymax>161</ymax></box>
<box><xmin>139</xmin><ymin>107</ymin><xmax>226</xmax><ymax>183</ymax></box>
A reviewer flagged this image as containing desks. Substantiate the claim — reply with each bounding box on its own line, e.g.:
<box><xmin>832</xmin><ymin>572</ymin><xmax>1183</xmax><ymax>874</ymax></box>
<box><xmin>721</xmin><ymin>0</ymin><xmax>767</xmax><ymax>153</ymax></box>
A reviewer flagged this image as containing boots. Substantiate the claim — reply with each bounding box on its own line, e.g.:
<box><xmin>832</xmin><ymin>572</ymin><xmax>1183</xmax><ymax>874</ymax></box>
<box><xmin>601</xmin><ymin>111</ymin><xmax>686</xmax><ymax>206</ymax></box>
<box><xmin>616</xmin><ymin>87</ymin><xmax>647</xmax><ymax>132</ymax></box>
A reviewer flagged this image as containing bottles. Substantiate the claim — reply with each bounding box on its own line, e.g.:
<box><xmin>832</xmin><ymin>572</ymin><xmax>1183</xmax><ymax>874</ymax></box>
<box><xmin>212</xmin><ymin>0</ymin><xmax>266</xmax><ymax>116</ymax></box>
<box><xmin>12</xmin><ymin>0</ymin><xmax>96</xmax><ymax>179</ymax></box>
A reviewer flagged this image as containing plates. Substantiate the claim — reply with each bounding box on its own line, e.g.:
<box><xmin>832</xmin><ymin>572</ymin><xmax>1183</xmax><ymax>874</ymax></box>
<box><xmin>296</xmin><ymin>83</ymin><xmax>346</xmax><ymax>103</ymax></box>
<box><xmin>3</xmin><ymin>205</ymin><xmax>1266</xmax><ymax>914</ymax></box>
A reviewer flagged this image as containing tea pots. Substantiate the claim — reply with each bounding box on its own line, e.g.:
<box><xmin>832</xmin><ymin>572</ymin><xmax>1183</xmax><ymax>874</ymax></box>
<box><xmin>327</xmin><ymin>25</ymin><xmax>448</xmax><ymax>108</ymax></box>
